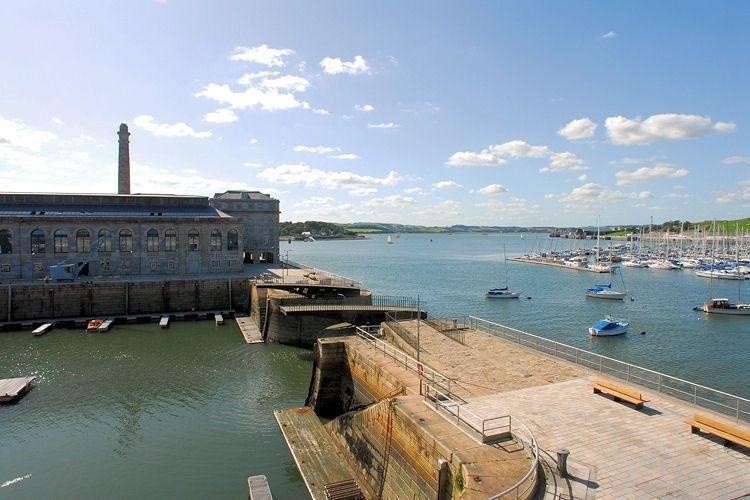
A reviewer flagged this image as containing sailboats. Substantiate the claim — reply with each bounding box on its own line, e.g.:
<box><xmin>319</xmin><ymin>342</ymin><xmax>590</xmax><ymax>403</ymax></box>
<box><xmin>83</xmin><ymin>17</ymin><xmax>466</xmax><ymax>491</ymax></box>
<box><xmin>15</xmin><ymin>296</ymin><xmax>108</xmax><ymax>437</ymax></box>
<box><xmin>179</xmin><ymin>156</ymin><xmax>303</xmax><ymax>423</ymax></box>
<box><xmin>509</xmin><ymin>214</ymin><xmax>750</xmax><ymax>299</ymax></box>
<box><xmin>702</xmin><ymin>246</ymin><xmax>750</xmax><ymax>315</ymax></box>
<box><xmin>485</xmin><ymin>242</ymin><xmax>526</xmax><ymax>299</ymax></box>
<box><xmin>387</xmin><ymin>234</ymin><xmax>394</xmax><ymax>244</ymax></box>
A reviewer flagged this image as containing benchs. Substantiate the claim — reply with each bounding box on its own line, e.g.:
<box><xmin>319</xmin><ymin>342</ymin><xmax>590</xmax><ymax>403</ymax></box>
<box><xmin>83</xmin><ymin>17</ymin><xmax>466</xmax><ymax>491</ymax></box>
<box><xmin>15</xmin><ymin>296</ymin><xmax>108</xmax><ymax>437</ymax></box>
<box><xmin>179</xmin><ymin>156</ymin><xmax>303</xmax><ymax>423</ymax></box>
<box><xmin>593</xmin><ymin>380</ymin><xmax>750</xmax><ymax>450</ymax></box>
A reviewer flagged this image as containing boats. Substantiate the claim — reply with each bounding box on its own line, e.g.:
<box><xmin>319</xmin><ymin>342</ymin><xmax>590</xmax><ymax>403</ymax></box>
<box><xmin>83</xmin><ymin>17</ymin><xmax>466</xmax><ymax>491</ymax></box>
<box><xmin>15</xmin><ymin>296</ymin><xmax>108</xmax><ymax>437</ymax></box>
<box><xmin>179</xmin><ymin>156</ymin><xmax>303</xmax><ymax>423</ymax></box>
<box><xmin>587</xmin><ymin>312</ymin><xmax>631</xmax><ymax>338</ymax></box>
<box><xmin>288</xmin><ymin>238</ymin><xmax>292</xmax><ymax>243</ymax></box>
<box><xmin>396</xmin><ymin>232</ymin><xmax>401</xmax><ymax>238</ymax></box>
<box><xmin>85</xmin><ymin>319</ymin><xmax>104</xmax><ymax>333</ymax></box>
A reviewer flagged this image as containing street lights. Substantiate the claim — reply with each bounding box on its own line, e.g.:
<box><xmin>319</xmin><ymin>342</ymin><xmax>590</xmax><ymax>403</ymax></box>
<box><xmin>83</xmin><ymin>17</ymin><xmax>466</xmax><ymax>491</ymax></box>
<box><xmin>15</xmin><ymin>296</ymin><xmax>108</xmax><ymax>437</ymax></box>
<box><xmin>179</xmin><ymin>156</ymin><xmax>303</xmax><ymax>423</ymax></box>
<box><xmin>284</xmin><ymin>249</ymin><xmax>292</xmax><ymax>276</ymax></box>
